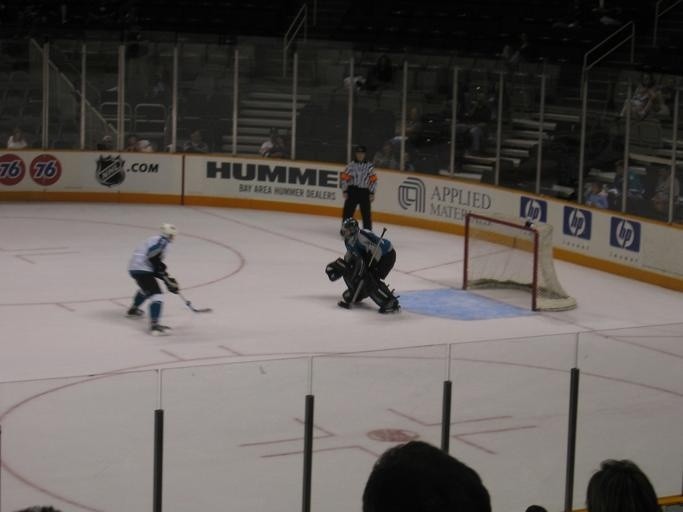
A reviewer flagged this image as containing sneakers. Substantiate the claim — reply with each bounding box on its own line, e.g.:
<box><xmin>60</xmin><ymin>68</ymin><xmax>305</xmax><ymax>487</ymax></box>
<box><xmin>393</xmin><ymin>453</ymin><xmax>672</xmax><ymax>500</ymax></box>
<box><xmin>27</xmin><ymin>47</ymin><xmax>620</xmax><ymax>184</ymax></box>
<box><xmin>346</xmin><ymin>289</ymin><xmax>363</xmax><ymax>301</ymax></box>
<box><xmin>637</xmin><ymin>110</ymin><xmax>647</xmax><ymax>120</ymax></box>
<box><xmin>150</xmin><ymin>321</ymin><xmax>172</xmax><ymax>332</ymax></box>
<box><xmin>378</xmin><ymin>296</ymin><xmax>399</xmax><ymax>312</ymax></box>
<box><xmin>128</xmin><ymin>306</ymin><xmax>144</xmax><ymax>315</ymax></box>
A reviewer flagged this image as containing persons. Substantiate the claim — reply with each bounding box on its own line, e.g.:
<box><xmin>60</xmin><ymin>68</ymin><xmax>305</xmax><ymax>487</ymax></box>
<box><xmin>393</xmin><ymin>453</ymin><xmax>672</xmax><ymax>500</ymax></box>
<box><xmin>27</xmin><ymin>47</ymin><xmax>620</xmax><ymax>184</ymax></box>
<box><xmin>340</xmin><ymin>144</ymin><xmax>377</xmax><ymax>235</ymax></box>
<box><xmin>126</xmin><ymin>222</ymin><xmax>178</xmax><ymax>332</ymax></box>
<box><xmin>361</xmin><ymin>440</ymin><xmax>493</xmax><ymax>507</ymax></box>
<box><xmin>6</xmin><ymin>34</ymin><xmax>680</xmax><ymax>221</ymax></box>
<box><xmin>324</xmin><ymin>217</ymin><xmax>398</xmax><ymax>310</ymax></box>
<box><xmin>584</xmin><ymin>459</ymin><xmax>664</xmax><ymax>505</ymax></box>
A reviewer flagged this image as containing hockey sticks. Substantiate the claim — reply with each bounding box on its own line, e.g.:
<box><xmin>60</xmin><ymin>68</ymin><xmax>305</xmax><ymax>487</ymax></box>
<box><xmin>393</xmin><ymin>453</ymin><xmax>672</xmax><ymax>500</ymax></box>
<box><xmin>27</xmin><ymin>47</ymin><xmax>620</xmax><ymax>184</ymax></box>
<box><xmin>163</xmin><ymin>275</ymin><xmax>212</xmax><ymax>313</ymax></box>
<box><xmin>338</xmin><ymin>227</ymin><xmax>388</xmax><ymax>310</ymax></box>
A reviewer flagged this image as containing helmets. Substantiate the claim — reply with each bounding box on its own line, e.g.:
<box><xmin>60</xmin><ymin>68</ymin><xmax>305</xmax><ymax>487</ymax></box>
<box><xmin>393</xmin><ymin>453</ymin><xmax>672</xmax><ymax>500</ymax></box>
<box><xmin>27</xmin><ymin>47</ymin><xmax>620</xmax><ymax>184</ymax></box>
<box><xmin>160</xmin><ymin>223</ymin><xmax>178</xmax><ymax>237</ymax></box>
<box><xmin>342</xmin><ymin>218</ymin><xmax>359</xmax><ymax>236</ymax></box>
<box><xmin>354</xmin><ymin>145</ymin><xmax>366</xmax><ymax>153</ymax></box>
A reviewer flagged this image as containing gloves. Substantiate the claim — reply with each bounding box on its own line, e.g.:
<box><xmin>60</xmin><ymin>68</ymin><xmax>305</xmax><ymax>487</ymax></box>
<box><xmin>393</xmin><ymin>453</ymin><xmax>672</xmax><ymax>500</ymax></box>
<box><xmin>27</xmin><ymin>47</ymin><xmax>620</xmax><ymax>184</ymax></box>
<box><xmin>154</xmin><ymin>261</ymin><xmax>167</xmax><ymax>275</ymax></box>
<box><xmin>165</xmin><ymin>278</ymin><xmax>178</xmax><ymax>294</ymax></box>
